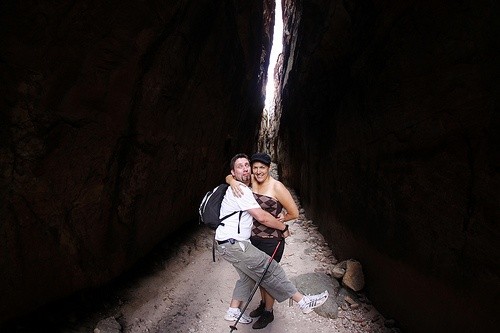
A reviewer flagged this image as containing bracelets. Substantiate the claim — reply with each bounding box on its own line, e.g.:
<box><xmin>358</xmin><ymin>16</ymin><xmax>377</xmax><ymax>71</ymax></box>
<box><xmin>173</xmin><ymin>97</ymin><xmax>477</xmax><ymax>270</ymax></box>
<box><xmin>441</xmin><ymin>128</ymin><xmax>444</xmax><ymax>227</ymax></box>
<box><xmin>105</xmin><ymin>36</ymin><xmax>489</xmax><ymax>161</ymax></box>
<box><xmin>281</xmin><ymin>225</ymin><xmax>288</xmax><ymax>232</ymax></box>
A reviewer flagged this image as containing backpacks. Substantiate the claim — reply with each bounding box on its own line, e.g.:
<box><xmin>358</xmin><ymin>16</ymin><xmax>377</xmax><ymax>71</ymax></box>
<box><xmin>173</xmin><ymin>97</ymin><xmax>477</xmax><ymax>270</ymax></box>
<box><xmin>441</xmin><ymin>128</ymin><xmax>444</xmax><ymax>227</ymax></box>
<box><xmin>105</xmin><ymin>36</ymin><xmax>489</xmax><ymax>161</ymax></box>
<box><xmin>199</xmin><ymin>184</ymin><xmax>243</xmax><ymax>230</ymax></box>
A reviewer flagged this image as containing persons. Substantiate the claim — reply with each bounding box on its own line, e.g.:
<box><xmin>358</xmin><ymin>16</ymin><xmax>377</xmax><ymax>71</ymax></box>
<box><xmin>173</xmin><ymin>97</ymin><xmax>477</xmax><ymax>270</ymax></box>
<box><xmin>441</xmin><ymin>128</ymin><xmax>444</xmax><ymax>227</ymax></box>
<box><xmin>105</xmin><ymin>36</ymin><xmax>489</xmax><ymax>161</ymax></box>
<box><xmin>214</xmin><ymin>153</ymin><xmax>328</xmax><ymax>323</ymax></box>
<box><xmin>225</xmin><ymin>154</ymin><xmax>299</xmax><ymax>328</ymax></box>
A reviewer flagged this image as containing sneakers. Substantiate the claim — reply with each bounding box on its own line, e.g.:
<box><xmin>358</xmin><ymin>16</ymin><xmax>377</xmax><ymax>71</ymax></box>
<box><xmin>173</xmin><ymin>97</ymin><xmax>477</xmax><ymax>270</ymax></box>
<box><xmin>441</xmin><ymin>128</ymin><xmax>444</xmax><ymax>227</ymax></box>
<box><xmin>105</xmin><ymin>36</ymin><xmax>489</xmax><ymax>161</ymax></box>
<box><xmin>299</xmin><ymin>290</ymin><xmax>328</xmax><ymax>314</ymax></box>
<box><xmin>223</xmin><ymin>310</ymin><xmax>253</xmax><ymax>324</ymax></box>
<box><xmin>252</xmin><ymin>309</ymin><xmax>274</xmax><ymax>329</ymax></box>
<box><xmin>249</xmin><ymin>300</ymin><xmax>266</xmax><ymax>317</ymax></box>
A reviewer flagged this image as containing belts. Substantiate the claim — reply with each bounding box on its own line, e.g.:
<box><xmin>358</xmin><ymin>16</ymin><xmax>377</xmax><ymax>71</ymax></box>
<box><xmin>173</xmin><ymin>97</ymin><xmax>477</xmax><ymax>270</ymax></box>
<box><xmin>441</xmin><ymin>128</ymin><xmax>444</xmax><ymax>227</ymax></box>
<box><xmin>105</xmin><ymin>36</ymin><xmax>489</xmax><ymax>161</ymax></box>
<box><xmin>218</xmin><ymin>239</ymin><xmax>229</xmax><ymax>244</ymax></box>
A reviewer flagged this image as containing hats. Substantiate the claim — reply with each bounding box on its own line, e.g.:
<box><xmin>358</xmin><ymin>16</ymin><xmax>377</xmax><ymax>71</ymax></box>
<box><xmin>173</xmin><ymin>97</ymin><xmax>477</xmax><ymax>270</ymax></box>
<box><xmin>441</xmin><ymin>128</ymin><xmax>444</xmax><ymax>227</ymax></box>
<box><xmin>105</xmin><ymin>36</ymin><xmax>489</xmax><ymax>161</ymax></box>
<box><xmin>250</xmin><ymin>153</ymin><xmax>271</xmax><ymax>166</ymax></box>
